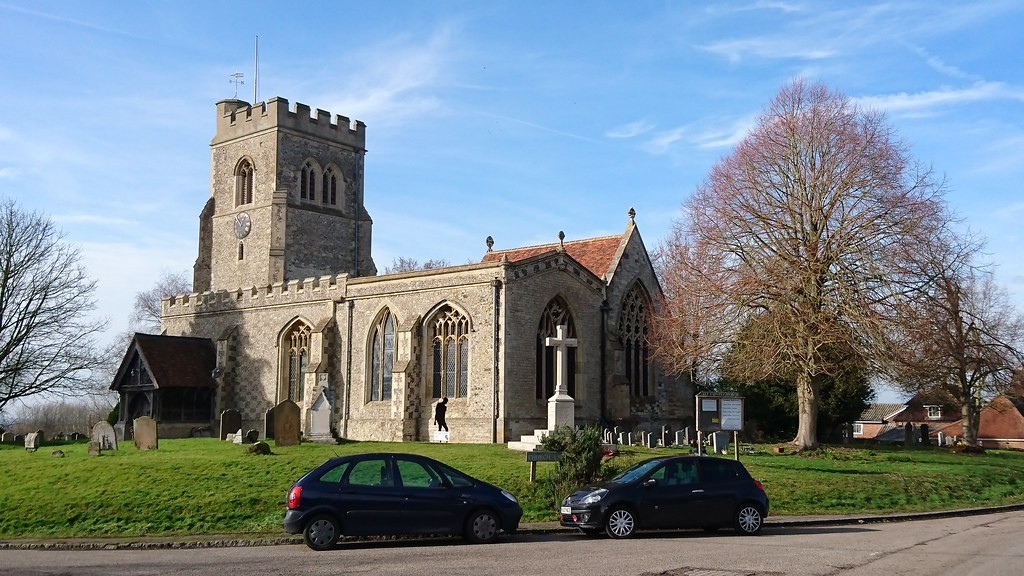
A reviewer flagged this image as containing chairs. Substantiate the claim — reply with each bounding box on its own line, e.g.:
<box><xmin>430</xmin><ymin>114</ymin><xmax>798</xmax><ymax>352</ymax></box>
<box><xmin>378</xmin><ymin>464</ymin><xmax>444</xmax><ymax>489</ymax></box>
<box><xmin>665</xmin><ymin>460</ymin><xmax>697</xmax><ymax>486</ymax></box>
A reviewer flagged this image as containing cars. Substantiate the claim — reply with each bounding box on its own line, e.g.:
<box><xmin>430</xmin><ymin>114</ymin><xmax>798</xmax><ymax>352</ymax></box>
<box><xmin>282</xmin><ymin>447</ymin><xmax>524</xmax><ymax>550</ymax></box>
<box><xmin>557</xmin><ymin>455</ymin><xmax>769</xmax><ymax>539</ymax></box>
